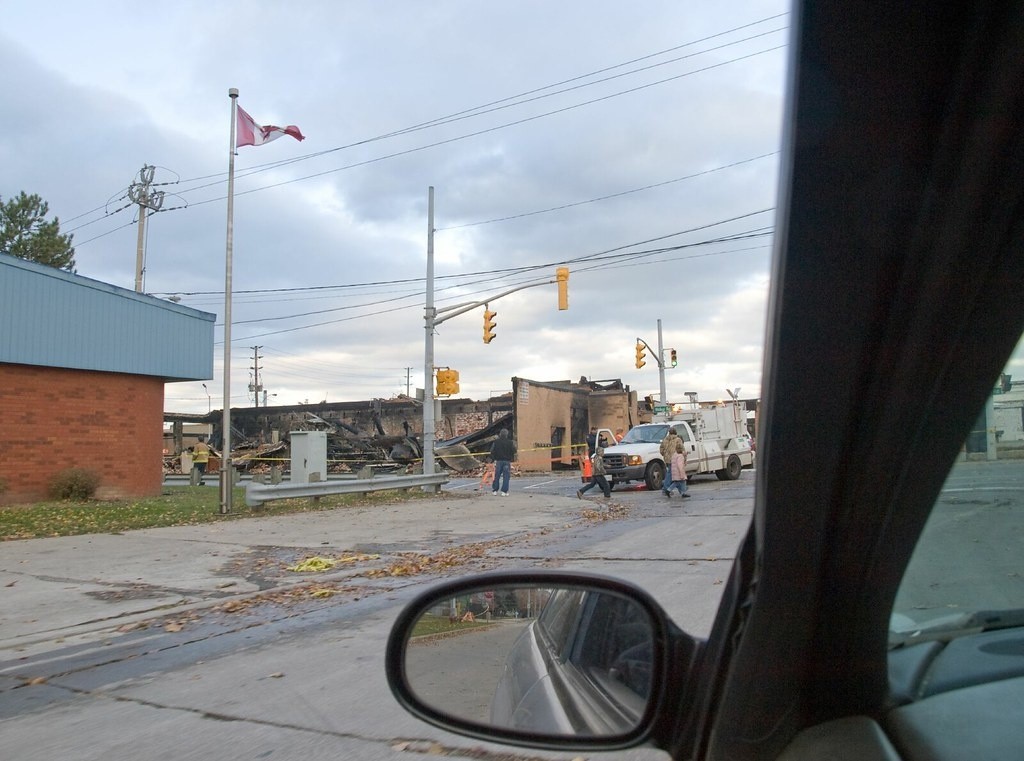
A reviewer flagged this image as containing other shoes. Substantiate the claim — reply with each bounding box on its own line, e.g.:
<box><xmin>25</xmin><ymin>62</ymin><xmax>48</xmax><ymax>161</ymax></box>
<box><xmin>662</xmin><ymin>490</ymin><xmax>674</xmax><ymax>495</ymax></box>
<box><xmin>577</xmin><ymin>489</ymin><xmax>582</xmax><ymax>500</ymax></box>
<box><xmin>501</xmin><ymin>491</ymin><xmax>510</xmax><ymax>497</ymax></box>
<box><xmin>682</xmin><ymin>493</ymin><xmax>691</xmax><ymax>497</ymax></box>
<box><xmin>604</xmin><ymin>495</ymin><xmax>612</xmax><ymax>500</ymax></box>
<box><xmin>665</xmin><ymin>491</ymin><xmax>671</xmax><ymax>498</ymax></box>
<box><xmin>491</xmin><ymin>490</ymin><xmax>498</xmax><ymax>495</ymax></box>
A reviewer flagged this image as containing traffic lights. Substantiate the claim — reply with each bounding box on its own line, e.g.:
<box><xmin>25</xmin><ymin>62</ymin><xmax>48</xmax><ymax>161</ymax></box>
<box><xmin>670</xmin><ymin>350</ymin><xmax>677</xmax><ymax>366</ymax></box>
<box><xmin>644</xmin><ymin>396</ymin><xmax>653</xmax><ymax>411</ymax></box>
<box><xmin>635</xmin><ymin>342</ymin><xmax>646</xmax><ymax>369</ymax></box>
<box><xmin>483</xmin><ymin>309</ymin><xmax>497</xmax><ymax>344</ymax></box>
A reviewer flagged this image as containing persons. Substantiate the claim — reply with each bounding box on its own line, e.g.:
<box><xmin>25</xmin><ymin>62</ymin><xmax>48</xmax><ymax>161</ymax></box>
<box><xmin>192</xmin><ymin>436</ymin><xmax>209</xmax><ymax>485</ymax></box>
<box><xmin>659</xmin><ymin>427</ymin><xmax>691</xmax><ymax>498</ymax></box>
<box><xmin>612</xmin><ymin>427</ymin><xmax>631</xmax><ymax>485</ymax></box>
<box><xmin>577</xmin><ymin>447</ymin><xmax>614</xmax><ymax>500</ymax></box>
<box><xmin>586</xmin><ymin>426</ymin><xmax>609</xmax><ymax>459</ymax></box>
<box><xmin>490</xmin><ymin>429</ymin><xmax>517</xmax><ymax>496</ymax></box>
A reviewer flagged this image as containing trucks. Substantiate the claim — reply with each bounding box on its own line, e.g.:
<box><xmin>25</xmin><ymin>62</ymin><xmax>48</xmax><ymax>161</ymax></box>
<box><xmin>590</xmin><ymin>401</ymin><xmax>752</xmax><ymax>491</ymax></box>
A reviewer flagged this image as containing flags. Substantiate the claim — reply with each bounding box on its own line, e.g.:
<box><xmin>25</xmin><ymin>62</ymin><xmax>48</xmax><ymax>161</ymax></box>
<box><xmin>236</xmin><ymin>104</ymin><xmax>306</xmax><ymax>148</ymax></box>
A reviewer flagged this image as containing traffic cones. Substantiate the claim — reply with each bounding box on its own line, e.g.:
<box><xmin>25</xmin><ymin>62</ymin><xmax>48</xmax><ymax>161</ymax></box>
<box><xmin>582</xmin><ymin>451</ymin><xmax>592</xmax><ymax>483</ymax></box>
